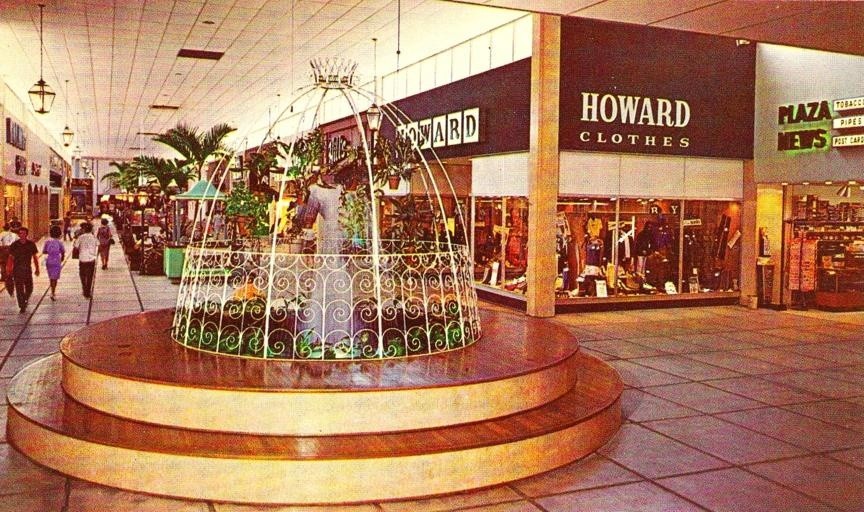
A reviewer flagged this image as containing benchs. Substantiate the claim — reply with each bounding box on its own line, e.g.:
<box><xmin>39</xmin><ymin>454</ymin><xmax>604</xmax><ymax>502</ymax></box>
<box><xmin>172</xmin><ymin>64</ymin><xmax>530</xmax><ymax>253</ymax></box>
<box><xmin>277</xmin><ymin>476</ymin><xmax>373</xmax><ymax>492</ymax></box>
<box><xmin>163</xmin><ymin>244</ymin><xmax>234</xmax><ymax>284</ymax></box>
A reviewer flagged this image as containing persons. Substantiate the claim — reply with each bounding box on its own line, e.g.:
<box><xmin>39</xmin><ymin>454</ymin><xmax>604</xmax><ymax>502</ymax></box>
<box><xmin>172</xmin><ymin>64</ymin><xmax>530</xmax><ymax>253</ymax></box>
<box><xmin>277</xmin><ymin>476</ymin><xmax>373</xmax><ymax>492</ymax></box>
<box><xmin>63</xmin><ymin>211</ymin><xmax>74</xmax><ymax>240</ymax></box>
<box><xmin>300</xmin><ymin>168</ymin><xmax>359</xmax><ymax>344</ymax></box>
<box><xmin>73</xmin><ymin>223</ymin><xmax>100</xmax><ymax>298</ymax></box>
<box><xmin>203</xmin><ymin>210</ymin><xmax>222</xmax><ymax>234</ymax></box>
<box><xmin>74</xmin><ymin>218</ymin><xmax>113</xmax><ymax>269</ymax></box>
<box><xmin>0</xmin><ymin>217</ymin><xmax>40</xmax><ymax>313</ymax></box>
<box><xmin>42</xmin><ymin>225</ymin><xmax>65</xmax><ymax>301</ymax></box>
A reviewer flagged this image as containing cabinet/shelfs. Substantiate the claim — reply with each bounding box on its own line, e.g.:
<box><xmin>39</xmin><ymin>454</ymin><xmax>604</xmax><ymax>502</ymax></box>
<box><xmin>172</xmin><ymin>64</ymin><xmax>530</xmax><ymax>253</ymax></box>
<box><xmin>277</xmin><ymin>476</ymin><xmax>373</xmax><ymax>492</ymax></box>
<box><xmin>816</xmin><ymin>239</ymin><xmax>863</xmax><ymax>309</ymax></box>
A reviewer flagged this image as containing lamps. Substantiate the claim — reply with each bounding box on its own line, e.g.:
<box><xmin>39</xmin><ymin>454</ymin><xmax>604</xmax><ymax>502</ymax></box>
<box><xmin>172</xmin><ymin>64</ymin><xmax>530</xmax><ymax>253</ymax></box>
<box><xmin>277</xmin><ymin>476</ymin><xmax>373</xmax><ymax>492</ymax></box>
<box><xmin>26</xmin><ymin>4</ymin><xmax>58</xmax><ymax>116</ymax></box>
<box><xmin>364</xmin><ymin>36</ymin><xmax>385</xmax><ymax>134</ymax></box>
<box><xmin>59</xmin><ymin>79</ymin><xmax>74</xmax><ymax>147</ymax></box>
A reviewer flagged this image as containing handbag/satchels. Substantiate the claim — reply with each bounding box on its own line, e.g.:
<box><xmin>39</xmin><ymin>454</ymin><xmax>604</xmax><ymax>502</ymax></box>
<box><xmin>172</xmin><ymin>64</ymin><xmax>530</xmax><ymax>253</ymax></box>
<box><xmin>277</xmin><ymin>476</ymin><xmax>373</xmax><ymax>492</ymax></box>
<box><xmin>110</xmin><ymin>239</ymin><xmax>114</xmax><ymax>244</ymax></box>
